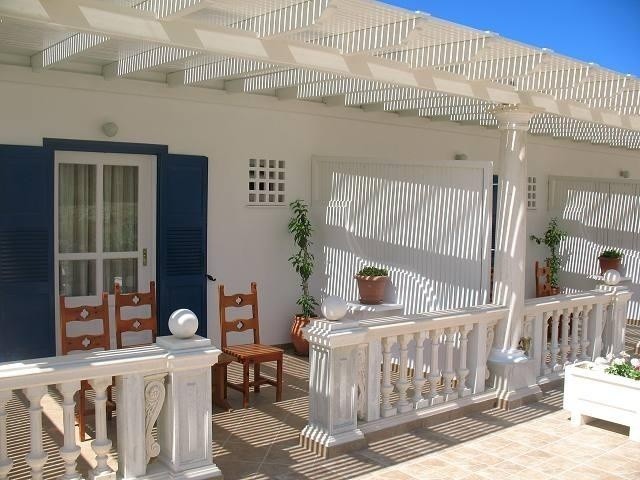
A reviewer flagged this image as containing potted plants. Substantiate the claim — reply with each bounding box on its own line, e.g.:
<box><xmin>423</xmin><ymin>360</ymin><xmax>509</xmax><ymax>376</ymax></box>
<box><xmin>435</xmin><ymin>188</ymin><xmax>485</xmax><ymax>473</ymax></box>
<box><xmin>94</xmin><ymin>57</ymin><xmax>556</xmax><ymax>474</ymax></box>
<box><xmin>597</xmin><ymin>250</ymin><xmax>622</xmax><ymax>276</ymax></box>
<box><xmin>528</xmin><ymin>217</ymin><xmax>567</xmax><ymax>297</ymax></box>
<box><xmin>288</xmin><ymin>196</ymin><xmax>323</xmax><ymax>357</ymax></box>
<box><xmin>354</xmin><ymin>266</ymin><xmax>389</xmax><ymax>305</ymax></box>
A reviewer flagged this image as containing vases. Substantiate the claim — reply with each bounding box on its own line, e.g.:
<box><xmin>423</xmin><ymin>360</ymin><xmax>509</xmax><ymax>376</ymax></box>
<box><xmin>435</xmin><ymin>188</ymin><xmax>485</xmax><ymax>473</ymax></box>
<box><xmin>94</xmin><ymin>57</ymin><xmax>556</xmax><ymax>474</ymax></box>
<box><xmin>564</xmin><ymin>361</ymin><xmax>640</xmax><ymax>443</ymax></box>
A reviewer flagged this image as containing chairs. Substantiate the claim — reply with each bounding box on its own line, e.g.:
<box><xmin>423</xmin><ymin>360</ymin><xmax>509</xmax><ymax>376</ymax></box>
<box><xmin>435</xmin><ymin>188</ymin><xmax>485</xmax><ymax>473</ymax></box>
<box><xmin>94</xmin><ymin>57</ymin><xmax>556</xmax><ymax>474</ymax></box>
<box><xmin>58</xmin><ymin>279</ymin><xmax>285</xmax><ymax>442</ymax></box>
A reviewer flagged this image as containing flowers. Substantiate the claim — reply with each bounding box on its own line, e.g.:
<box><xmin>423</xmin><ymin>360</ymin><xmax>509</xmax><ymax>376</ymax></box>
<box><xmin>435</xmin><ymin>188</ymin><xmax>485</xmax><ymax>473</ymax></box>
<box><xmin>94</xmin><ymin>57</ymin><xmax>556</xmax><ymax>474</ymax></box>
<box><xmin>588</xmin><ymin>350</ymin><xmax>640</xmax><ymax>378</ymax></box>
